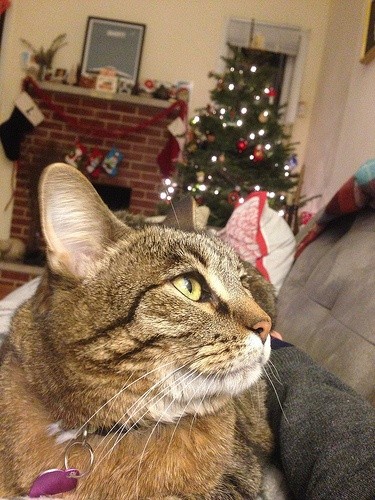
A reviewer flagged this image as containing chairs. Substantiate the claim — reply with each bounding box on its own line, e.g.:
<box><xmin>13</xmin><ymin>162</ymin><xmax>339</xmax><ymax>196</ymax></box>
<box><xmin>274</xmin><ymin>161</ymin><xmax>375</xmax><ymax>414</ymax></box>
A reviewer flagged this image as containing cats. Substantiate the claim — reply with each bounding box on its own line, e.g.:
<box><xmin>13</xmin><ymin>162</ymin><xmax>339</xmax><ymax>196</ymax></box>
<box><xmin>0</xmin><ymin>160</ymin><xmax>278</xmax><ymax>500</ymax></box>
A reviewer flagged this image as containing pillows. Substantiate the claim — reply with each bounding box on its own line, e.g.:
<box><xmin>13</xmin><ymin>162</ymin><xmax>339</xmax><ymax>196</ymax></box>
<box><xmin>216</xmin><ymin>191</ymin><xmax>297</xmax><ymax>298</ymax></box>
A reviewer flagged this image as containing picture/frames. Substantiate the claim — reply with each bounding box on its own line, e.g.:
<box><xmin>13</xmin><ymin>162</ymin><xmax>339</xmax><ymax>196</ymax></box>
<box><xmin>79</xmin><ymin>16</ymin><xmax>146</xmax><ymax>90</ymax></box>
